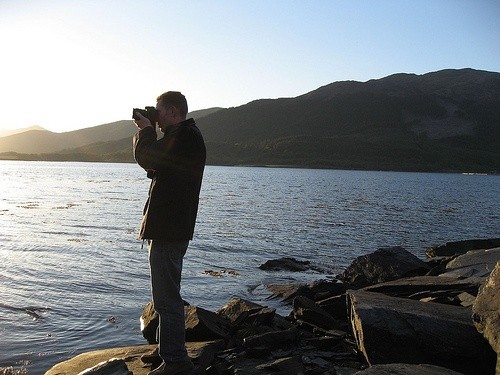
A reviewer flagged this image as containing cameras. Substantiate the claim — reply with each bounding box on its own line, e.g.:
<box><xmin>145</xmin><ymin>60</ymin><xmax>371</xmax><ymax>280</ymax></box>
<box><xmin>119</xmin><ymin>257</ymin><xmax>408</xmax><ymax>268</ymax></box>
<box><xmin>132</xmin><ymin>106</ymin><xmax>158</xmax><ymax>125</ymax></box>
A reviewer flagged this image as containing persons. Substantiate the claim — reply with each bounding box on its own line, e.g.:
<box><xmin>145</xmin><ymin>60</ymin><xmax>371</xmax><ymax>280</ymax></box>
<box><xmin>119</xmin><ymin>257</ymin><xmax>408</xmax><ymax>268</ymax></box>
<box><xmin>132</xmin><ymin>91</ymin><xmax>206</xmax><ymax>375</ymax></box>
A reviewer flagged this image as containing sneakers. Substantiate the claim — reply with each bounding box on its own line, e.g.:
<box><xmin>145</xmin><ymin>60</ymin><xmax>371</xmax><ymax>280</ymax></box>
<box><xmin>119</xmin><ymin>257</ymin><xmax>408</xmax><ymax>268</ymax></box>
<box><xmin>141</xmin><ymin>347</ymin><xmax>188</xmax><ymax>363</ymax></box>
<box><xmin>148</xmin><ymin>356</ymin><xmax>193</xmax><ymax>375</ymax></box>
